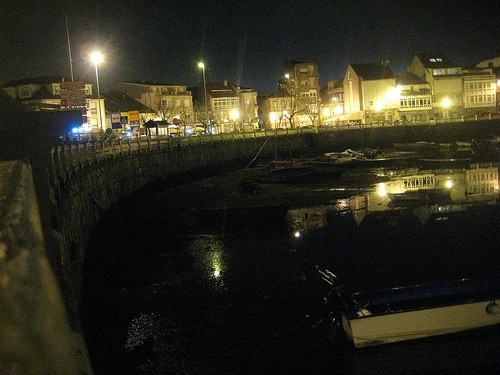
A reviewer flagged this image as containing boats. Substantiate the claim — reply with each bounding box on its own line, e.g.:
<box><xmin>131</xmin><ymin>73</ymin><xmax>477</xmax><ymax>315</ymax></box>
<box><xmin>317</xmin><ymin>268</ymin><xmax>500</xmax><ymax>349</ymax></box>
<box><xmin>151</xmin><ymin>133</ymin><xmax>500</xmax><ymax>243</ymax></box>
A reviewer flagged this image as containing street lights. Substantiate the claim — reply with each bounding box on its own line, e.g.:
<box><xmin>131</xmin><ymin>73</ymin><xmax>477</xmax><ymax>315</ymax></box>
<box><xmin>284</xmin><ymin>72</ymin><xmax>294</xmax><ymax>115</ymax></box>
<box><xmin>89</xmin><ymin>51</ymin><xmax>105</xmax><ymax>136</ymax></box>
<box><xmin>197</xmin><ymin>62</ymin><xmax>210</xmax><ymax>133</ymax></box>
